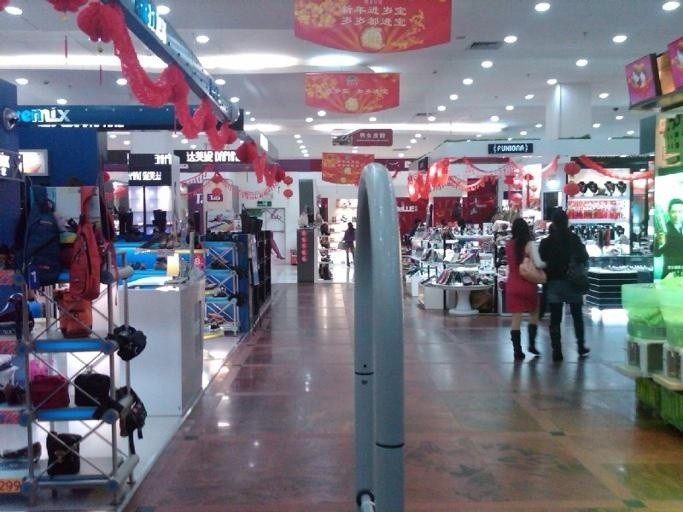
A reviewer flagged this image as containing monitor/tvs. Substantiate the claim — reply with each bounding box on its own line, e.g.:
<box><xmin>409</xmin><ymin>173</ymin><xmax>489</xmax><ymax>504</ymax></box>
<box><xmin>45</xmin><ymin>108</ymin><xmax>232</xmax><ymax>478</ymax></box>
<box><xmin>17</xmin><ymin>148</ymin><xmax>49</xmax><ymax>177</ymax></box>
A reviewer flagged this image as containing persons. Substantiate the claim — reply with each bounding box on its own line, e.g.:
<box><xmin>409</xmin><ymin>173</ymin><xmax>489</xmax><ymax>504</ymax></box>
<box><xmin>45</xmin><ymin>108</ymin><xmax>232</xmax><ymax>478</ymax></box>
<box><xmin>539</xmin><ymin>212</ymin><xmax>589</xmax><ymax>360</ymax></box>
<box><xmin>650</xmin><ymin>199</ymin><xmax>683</xmax><ymax>279</ymax></box>
<box><xmin>272</xmin><ymin>240</ymin><xmax>286</xmax><ymax>259</ymax></box>
<box><xmin>342</xmin><ymin>223</ymin><xmax>356</xmax><ymax>266</ymax></box>
<box><xmin>506</xmin><ymin>219</ymin><xmax>547</xmax><ymax>359</ymax></box>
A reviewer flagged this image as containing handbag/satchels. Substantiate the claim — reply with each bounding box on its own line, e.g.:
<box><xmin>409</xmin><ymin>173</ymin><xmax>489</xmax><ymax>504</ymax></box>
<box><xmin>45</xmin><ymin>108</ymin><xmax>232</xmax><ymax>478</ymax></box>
<box><xmin>22</xmin><ymin>185</ymin><xmax>61</xmax><ymax>290</ymax></box>
<box><xmin>53</xmin><ymin>285</ymin><xmax>94</xmax><ymax>339</ymax></box>
<box><xmin>45</xmin><ymin>431</ymin><xmax>81</xmax><ymax>477</ymax></box>
<box><xmin>518</xmin><ymin>257</ymin><xmax>549</xmax><ymax>284</ymax></box>
<box><xmin>106</xmin><ymin>323</ymin><xmax>149</xmax><ymax>362</ymax></box>
<box><xmin>31</xmin><ymin>375</ymin><xmax>70</xmax><ymax>409</ymax></box>
<box><xmin>115</xmin><ymin>386</ymin><xmax>148</xmax><ymax>455</ymax></box>
<box><xmin>69</xmin><ymin>186</ymin><xmax>101</xmax><ymax>300</ymax></box>
<box><xmin>74</xmin><ymin>373</ymin><xmax>112</xmax><ymax>408</ymax></box>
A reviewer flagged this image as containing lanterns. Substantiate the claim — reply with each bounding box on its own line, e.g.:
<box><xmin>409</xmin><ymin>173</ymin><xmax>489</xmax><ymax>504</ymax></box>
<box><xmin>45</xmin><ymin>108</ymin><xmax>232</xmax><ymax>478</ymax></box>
<box><xmin>218</xmin><ymin>129</ymin><xmax>237</xmax><ymax>144</ymax></box>
<box><xmin>564</xmin><ymin>181</ymin><xmax>579</xmax><ymax>198</ymax></box>
<box><xmin>212</xmin><ymin>188</ymin><xmax>222</xmax><ymax>195</ymax></box>
<box><xmin>77</xmin><ymin>2</ymin><xmax>118</xmax><ymax>85</ymax></box>
<box><xmin>48</xmin><ymin>0</ymin><xmax>86</xmax><ymax>56</ymax></box>
<box><xmin>565</xmin><ymin>162</ymin><xmax>580</xmax><ymax>179</ymax></box>
<box><xmin>236</xmin><ymin>142</ymin><xmax>257</xmax><ymax>181</ymax></box>
<box><xmin>168</xmin><ymin>80</ymin><xmax>188</xmax><ymax>134</ymax></box>
<box><xmin>283</xmin><ymin>190</ymin><xmax>293</xmax><ymax>199</ymax></box>
<box><xmin>212</xmin><ymin>176</ymin><xmax>223</xmax><ymax>183</ymax></box>
<box><xmin>504</xmin><ymin>169</ymin><xmax>536</xmax><ymax>209</ymax></box>
<box><xmin>271</xmin><ymin>167</ymin><xmax>285</xmax><ymax>182</ymax></box>
<box><xmin>283</xmin><ymin>176</ymin><xmax>293</xmax><ymax>186</ymax></box>
<box><xmin>0</xmin><ymin>0</ymin><xmax>10</xmax><ymax>11</ymax></box>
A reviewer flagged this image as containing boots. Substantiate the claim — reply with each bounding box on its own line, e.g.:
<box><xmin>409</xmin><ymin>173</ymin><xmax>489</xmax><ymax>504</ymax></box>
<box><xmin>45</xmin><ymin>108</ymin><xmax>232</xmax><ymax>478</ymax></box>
<box><xmin>113</xmin><ymin>209</ymin><xmax>202</xmax><ymax>287</ymax></box>
<box><xmin>319</xmin><ymin>222</ymin><xmax>333</xmax><ymax>282</ymax></box>
<box><xmin>509</xmin><ymin>328</ymin><xmax>525</xmax><ymax>360</ymax></box>
<box><xmin>527</xmin><ymin>323</ymin><xmax>541</xmax><ymax>356</ymax></box>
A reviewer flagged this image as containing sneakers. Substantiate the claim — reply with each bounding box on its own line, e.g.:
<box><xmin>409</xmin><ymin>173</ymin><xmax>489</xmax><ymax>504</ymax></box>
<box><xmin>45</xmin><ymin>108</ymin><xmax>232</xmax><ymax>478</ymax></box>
<box><xmin>577</xmin><ymin>344</ymin><xmax>590</xmax><ymax>358</ymax></box>
<box><xmin>551</xmin><ymin>346</ymin><xmax>566</xmax><ymax>363</ymax></box>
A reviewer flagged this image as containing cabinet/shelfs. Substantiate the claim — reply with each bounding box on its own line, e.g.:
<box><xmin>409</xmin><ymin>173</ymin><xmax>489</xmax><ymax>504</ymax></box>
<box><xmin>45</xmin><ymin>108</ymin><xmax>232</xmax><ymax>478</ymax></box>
<box><xmin>399</xmin><ymin>156</ymin><xmax>655</xmax><ymax>320</ymax></box>
<box><xmin>0</xmin><ymin>223</ymin><xmax>276</xmax><ymax>511</ymax></box>
<box><xmin>312</xmin><ymin>196</ymin><xmax>358</xmax><ymax>268</ymax></box>
